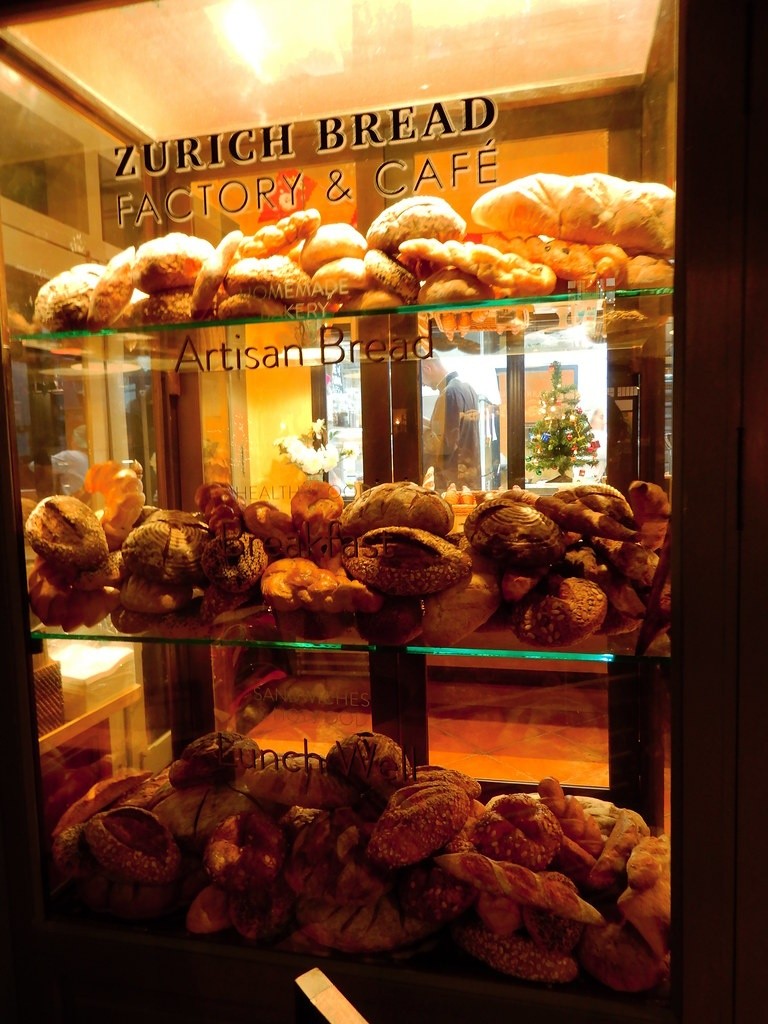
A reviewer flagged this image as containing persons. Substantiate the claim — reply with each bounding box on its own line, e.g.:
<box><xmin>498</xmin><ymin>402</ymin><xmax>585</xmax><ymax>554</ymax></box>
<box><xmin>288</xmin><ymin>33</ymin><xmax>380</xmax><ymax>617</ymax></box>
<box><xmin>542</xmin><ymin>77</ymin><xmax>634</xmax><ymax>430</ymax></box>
<box><xmin>26</xmin><ymin>425</ymin><xmax>87</xmax><ymax>494</ymax></box>
<box><xmin>422</xmin><ymin>352</ymin><xmax>482</xmax><ymax>490</ymax></box>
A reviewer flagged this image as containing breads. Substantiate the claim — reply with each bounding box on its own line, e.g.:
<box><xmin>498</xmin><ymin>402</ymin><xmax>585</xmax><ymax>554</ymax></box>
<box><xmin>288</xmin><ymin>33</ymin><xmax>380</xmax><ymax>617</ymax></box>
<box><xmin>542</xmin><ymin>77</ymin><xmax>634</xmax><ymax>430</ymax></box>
<box><xmin>28</xmin><ymin>172</ymin><xmax>676</xmax><ymax>346</ymax></box>
<box><xmin>24</xmin><ymin>458</ymin><xmax>679</xmax><ymax>646</ymax></box>
<box><xmin>46</xmin><ymin>727</ymin><xmax>674</xmax><ymax>998</ymax></box>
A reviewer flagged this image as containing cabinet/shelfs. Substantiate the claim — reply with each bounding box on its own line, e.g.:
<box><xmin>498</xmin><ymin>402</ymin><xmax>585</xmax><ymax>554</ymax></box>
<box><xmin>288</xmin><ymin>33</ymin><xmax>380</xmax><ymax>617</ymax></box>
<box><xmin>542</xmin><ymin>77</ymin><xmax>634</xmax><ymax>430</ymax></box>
<box><xmin>0</xmin><ymin>0</ymin><xmax>751</xmax><ymax>1024</ymax></box>
